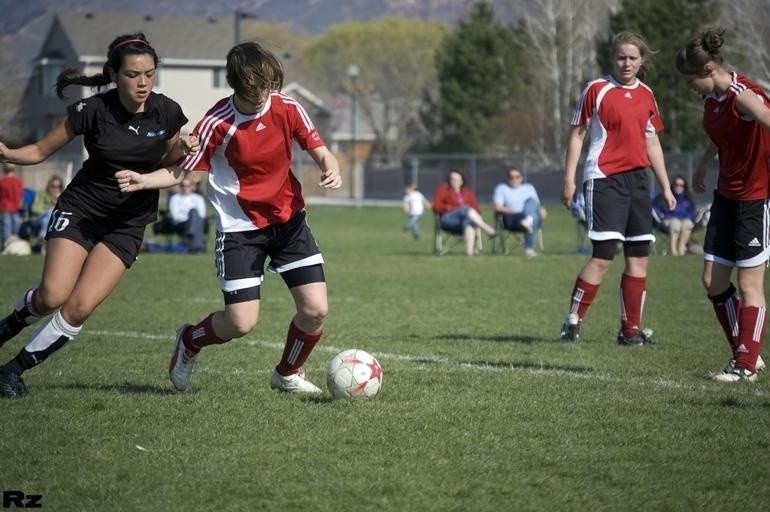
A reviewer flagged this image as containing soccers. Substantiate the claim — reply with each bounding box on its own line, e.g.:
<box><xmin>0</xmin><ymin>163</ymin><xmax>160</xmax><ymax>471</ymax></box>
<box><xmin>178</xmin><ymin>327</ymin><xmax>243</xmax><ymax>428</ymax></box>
<box><xmin>328</xmin><ymin>348</ymin><xmax>382</xmax><ymax>400</ymax></box>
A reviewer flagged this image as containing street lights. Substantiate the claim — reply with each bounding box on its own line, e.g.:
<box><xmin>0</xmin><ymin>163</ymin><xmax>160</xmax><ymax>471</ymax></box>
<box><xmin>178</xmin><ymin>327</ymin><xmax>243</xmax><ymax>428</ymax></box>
<box><xmin>346</xmin><ymin>59</ymin><xmax>362</xmax><ymax>200</ymax></box>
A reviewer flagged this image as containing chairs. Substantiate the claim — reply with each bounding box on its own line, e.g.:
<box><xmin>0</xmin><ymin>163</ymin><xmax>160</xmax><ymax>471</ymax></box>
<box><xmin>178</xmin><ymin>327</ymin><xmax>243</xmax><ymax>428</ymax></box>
<box><xmin>434</xmin><ymin>212</ymin><xmax>483</xmax><ymax>256</ymax></box>
<box><xmin>655</xmin><ymin>224</ymin><xmax>702</xmax><ymax>257</ymax></box>
<box><xmin>24</xmin><ymin>190</ymin><xmax>38</xmax><ymax>244</ymax></box>
<box><xmin>492</xmin><ymin>214</ymin><xmax>544</xmax><ymax>257</ymax></box>
<box><xmin>576</xmin><ymin>221</ymin><xmax>592</xmax><ymax>254</ymax></box>
<box><xmin>157</xmin><ymin>190</ymin><xmax>209</xmax><ymax>254</ymax></box>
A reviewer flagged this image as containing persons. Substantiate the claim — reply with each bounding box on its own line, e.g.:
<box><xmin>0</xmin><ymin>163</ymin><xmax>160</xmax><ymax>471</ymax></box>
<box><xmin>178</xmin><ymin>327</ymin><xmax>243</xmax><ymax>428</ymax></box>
<box><xmin>432</xmin><ymin>169</ymin><xmax>499</xmax><ymax>255</ymax></box>
<box><xmin>492</xmin><ymin>167</ymin><xmax>547</xmax><ymax>258</ymax></box>
<box><xmin>675</xmin><ymin>27</ymin><xmax>770</xmax><ymax>383</ymax></box>
<box><xmin>0</xmin><ymin>163</ymin><xmax>208</xmax><ymax>256</ymax></box>
<box><xmin>561</xmin><ymin>33</ymin><xmax>678</xmax><ymax>347</ymax></box>
<box><xmin>0</xmin><ymin>32</ymin><xmax>201</xmax><ymax>405</ymax></box>
<box><xmin>403</xmin><ymin>178</ymin><xmax>430</xmax><ymax>240</ymax></box>
<box><xmin>652</xmin><ymin>175</ymin><xmax>697</xmax><ymax>255</ymax></box>
<box><xmin>116</xmin><ymin>42</ymin><xmax>344</xmax><ymax>393</ymax></box>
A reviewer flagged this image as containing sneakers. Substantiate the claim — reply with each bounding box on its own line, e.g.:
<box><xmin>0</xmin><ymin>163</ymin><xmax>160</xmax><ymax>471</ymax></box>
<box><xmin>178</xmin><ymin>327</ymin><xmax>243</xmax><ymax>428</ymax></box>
<box><xmin>722</xmin><ymin>352</ymin><xmax>767</xmax><ymax>376</ymax></box>
<box><xmin>615</xmin><ymin>328</ymin><xmax>655</xmax><ymax>347</ymax></box>
<box><xmin>0</xmin><ymin>311</ymin><xmax>23</xmax><ymax>350</ymax></box>
<box><xmin>268</xmin><ymin>366</ymin><xmax>323</xmax><ymax>394</ymax></box>
<box><xmin>711</xmin><ymin>369</ymin><xmax>760</xmax><ymax>384</ymax></box>
<box><xmin>167</xmin><ymin>318</ymin><xmax>199</xmax><ymax>393</ymax></box>
<box><xmin>558</xmin><ymin>310</ymin><xmax>582</xmax><ymax>343</ymax></box>
<box><xmin>0</xmin><ymin>364</ymin><xmax>29</xmax><ymax>397</ymax></box>
